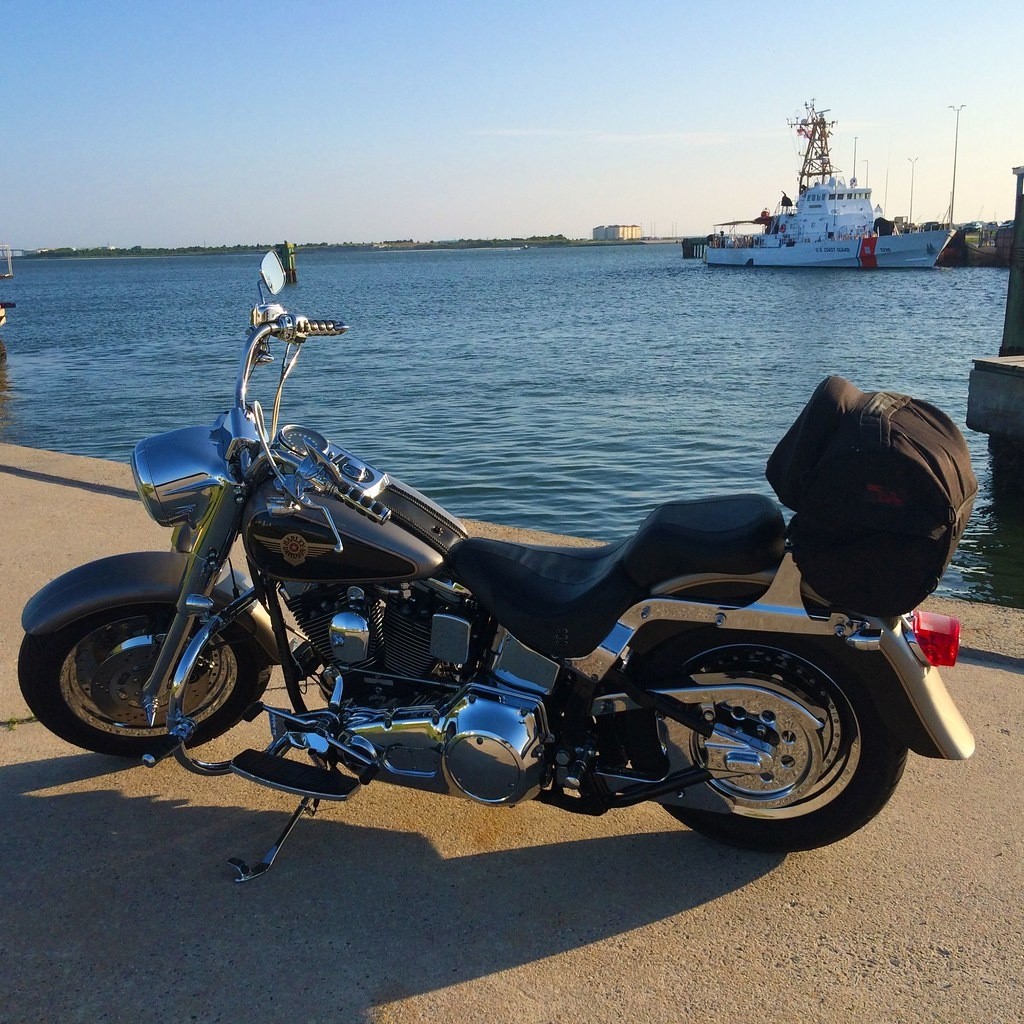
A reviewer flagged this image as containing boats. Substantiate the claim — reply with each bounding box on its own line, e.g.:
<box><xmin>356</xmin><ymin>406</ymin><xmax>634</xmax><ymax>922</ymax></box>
<box><xmin>699</xmin><ymin>97</ymin><xmax>958</xmax><ymax>268</ymax></box>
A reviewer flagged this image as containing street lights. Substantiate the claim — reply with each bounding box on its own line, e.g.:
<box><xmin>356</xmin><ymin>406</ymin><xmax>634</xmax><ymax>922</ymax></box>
<box><xmin>948</xmin><ymin>104</ymin><xmax>967</xmax><ymax>231</ymax></box>
<box><xmin>852</xmin><ymin>136</ymin><xmax>859</xmax><ymax>177</ymax></box>
<box><xmin>907</xmin><ymin>156</ymin><xmax>920</xmax><ymax>224</ymax></box>
<box><xmin>863</xmin><ymin>159</ymin><xmax>869</xmax><ymax>189</ymax></box>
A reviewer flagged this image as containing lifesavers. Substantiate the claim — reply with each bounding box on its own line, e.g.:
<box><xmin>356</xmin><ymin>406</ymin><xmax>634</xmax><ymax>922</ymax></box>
<box><xmin>780</xmin><ymin>224</ymin><xmax>786</xmax><ymax>233</ymax></box>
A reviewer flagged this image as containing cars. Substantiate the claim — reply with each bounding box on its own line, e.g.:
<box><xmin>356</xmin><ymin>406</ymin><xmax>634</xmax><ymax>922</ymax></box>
<box><xmin>963</xmin><ymin>219</ymin><xmax>1015</xmax><ymax>231</ymax></box>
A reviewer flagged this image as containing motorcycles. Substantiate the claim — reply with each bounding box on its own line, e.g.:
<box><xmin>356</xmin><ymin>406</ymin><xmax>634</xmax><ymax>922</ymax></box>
<box><xmin>15</xmin><ymin>248</ymin><xmax>979</xmax><ymax>885</ymax></box>
